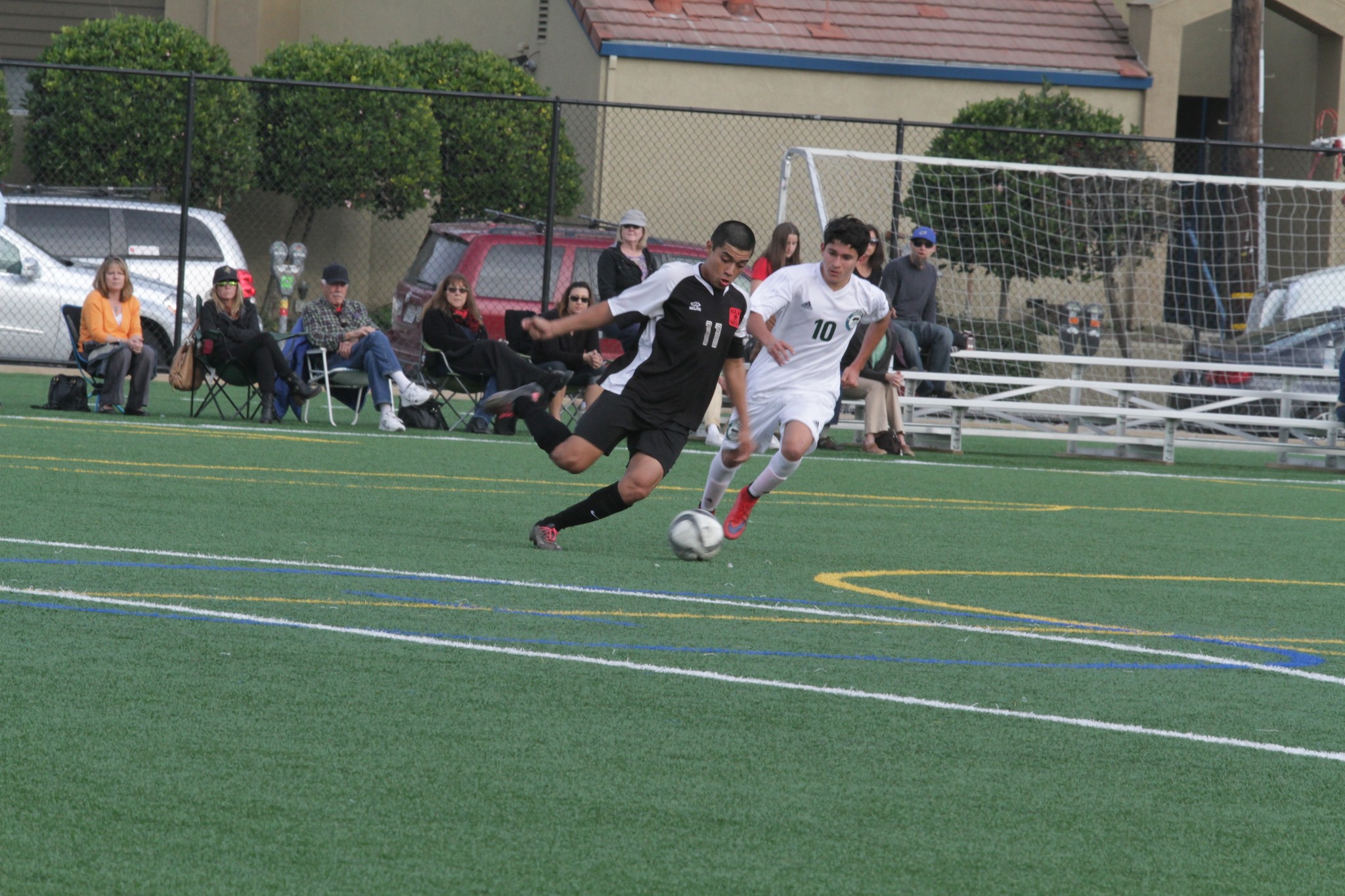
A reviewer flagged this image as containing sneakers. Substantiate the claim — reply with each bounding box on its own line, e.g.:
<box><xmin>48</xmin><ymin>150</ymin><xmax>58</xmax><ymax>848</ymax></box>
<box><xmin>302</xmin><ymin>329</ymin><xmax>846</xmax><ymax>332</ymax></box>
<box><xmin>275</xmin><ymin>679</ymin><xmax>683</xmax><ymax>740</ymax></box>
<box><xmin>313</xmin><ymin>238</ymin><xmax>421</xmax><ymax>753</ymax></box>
<box><xmin>529</xmin><ymin>521</ymin><xmax>562</xmax><ymax>551</ymax></box>
<box><xmin>686</xmin><ymin>424</ymin><xmax>708</xmax><ymax>440</ymax></box>
<box><xmin>483</xmin><ymin>383</ymin><xmax>542</xmax><ymax>419</ymax></box>
<box><xmin>724</xmin><ymin>482</ymin><xmax>760</xmax><ymax>540</ymax></box>
<box><xmin>705</xmin><ymin>433</ymin><xmax>725</xmax><ymax>447</ymax></box>
<box><xmin>398</xmin><ymin>383</ymin><xmax>433</xmax><ymax>407</ymax></box>
<box><xmin>699</xmin><ymin>502</ymin><xmax>715</xmax><ymax>515</ymax></box>
<box><xmin>379</xmin><ymin>412</ymin><xmax>406</xmax><ymax>432</ymax></box>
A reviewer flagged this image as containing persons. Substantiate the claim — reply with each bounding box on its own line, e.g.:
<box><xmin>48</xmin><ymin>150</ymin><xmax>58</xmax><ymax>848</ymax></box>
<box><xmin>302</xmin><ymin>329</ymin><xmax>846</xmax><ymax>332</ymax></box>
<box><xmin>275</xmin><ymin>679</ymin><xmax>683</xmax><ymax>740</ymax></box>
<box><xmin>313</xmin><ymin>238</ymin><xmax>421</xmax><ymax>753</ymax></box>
<box><xmin>686</xmin><ymin>378</ymin><xmax>730</xmax><ymax>447</ymax></box>
<box><xmin>876</xmin><ymin>223</ymin><xmax>956</xmax><ymax>406</ymax></box>
<box><xmin>816</xmin><ymin>377</ymin><xmax>840</xmax><ymax>453</ymax></box>
<box><xmin>846</xmin><ymin>297</ymin><xmax>917</xmax><ymax>456</ymax></box>
<box><xmin>749</xmin><ymin>223</ymin><xmax>805</xmax><ymax>358</ymax></box>
<box><xmin>696</xmin><ymin>208</ymin><xmax>890</xmax><ymax>546</ymax></box>
<box><xmin>515</xmin><ymin>280</ymin><xmax>609</xmax><ymax>433</ymax></box>
<box><xmin>78</xmin><ymin>254</ymin><xmax>156</xmax><ymax>416</ymax></box>
<box><xmin>596</xmin><ymin>209</ymin><xmax>659</xmax><ymax>362</ymax></box>
<box><xmin>200</xmin><ymin>266</ymin><xmax>323</xmax><ymax>423</ymax></box>
<box><xmin>480</xmin><ymin>221</ymin><xmax>762</xmax><ymax>550</ymax></box>
<box><xmin>851</xmin><ymin>222</ymin><xmax>887</xmax><ymax>293</ymax></box>
<box><xmin>418</xmin><ymin>273</ymin><xmax>574</xmax><ymax>434</ymax></box>
<box><xmin>298</xmin><ymin>262</ymin><xmax>434</xmax><ymax>431</ymax></box>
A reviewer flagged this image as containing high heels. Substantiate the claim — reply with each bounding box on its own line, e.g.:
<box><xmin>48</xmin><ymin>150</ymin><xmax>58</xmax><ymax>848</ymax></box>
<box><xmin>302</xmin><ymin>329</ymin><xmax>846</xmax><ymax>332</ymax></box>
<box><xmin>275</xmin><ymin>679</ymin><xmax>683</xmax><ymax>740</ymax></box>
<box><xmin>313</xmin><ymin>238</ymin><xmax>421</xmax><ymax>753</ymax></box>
<box><xmin>260</xmin><ymin>392</ymin><xmax>274</xmax><ymax>424</ymax></box>
<box><xmin>285</xmin><ymin>372</ymin><xmax>322</xmax><ymax>407</ymax></box>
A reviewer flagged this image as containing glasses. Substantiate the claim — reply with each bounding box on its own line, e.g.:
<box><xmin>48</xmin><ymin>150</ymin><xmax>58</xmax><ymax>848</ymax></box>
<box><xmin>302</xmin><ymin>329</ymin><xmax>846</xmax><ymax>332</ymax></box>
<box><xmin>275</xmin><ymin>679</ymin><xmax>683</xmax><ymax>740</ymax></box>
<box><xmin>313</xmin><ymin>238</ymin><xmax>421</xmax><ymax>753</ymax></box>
<box><xmin>912</xmin><ymin>239</ymin><xmax>934</xmax><ymax>248</ymax></box>
<box><xmin>870</xmin><ymin>237</ymin><xmax>879</xmax><ymax>246</ymax></box>
<box><xmin>569</xmin><ymin>296</ymin><xmax>588</xmax><ymax>303</ymax></box>
<box><xmin>446</xmin><ymin>287</ymin><xmax>468</xmax><ymax>294</ymax></box>
<box><xmin>216</xmin><ymin>281</ymin><xmax>238</xmax><ymax>286</ymax></box>
<box><xmin>623</xmin><ymin>224</ymin><xmax>640</xmax><ymax>229</ymax></box>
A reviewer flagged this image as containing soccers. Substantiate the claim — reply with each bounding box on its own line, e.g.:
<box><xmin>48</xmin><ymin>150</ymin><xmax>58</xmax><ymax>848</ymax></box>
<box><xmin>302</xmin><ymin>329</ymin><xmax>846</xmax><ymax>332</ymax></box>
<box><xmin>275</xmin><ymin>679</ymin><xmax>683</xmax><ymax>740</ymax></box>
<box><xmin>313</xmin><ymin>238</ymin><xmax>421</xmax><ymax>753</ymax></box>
<box><xmin>668</xmin><ymin>508</ymin><xmax>725</xmax><ymax>561</ymax></box>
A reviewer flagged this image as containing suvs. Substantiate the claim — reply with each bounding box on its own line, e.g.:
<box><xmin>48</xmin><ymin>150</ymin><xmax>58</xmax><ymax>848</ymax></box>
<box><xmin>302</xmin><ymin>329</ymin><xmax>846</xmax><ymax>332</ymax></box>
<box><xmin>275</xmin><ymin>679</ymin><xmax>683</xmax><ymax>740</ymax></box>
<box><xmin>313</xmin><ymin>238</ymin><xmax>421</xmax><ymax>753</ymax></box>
<box><xmin>1246</xmin><ymin>264</ymin><xmax>1345</xmax><ymax>331</ymax></box>
<box><xmin>384</xmin><ymin>208</ymin><xmax>754</xmax><ymax>395</ymax></box>
<box><xmin>0</xmin><ymin>182</ymin><xmax>263</xmax><ymax>374</ymax></box>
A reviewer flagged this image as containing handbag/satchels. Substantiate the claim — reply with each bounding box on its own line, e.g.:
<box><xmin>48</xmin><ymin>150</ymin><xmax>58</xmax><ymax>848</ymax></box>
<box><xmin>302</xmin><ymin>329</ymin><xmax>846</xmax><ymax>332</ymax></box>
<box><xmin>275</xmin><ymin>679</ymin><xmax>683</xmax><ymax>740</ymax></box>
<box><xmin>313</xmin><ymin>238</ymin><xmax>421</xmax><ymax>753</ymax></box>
<box><xmin>48</xmin><ymin>374</ymin><xmax>88</xmax><ymax>412</ymax></box>
<box><xmin>877</xmin><ymin>430</ymin><xmax>901</xmax><ymax>456</ymax></box>
<box><xmin>169</xmin><ymin>317</ymin><xmax>203</xmax><ymax>390</ymax></box>
<box><xmin>398</xmin><ymin>394</ymin><xmax>450</xmax><ymax>431</ymax></box>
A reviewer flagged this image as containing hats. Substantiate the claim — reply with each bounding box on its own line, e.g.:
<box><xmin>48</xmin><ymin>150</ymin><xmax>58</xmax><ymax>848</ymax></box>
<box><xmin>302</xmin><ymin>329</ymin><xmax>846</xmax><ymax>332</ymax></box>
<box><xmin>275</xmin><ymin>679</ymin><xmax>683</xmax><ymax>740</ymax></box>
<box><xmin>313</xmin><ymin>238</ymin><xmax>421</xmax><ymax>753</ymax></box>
<box><xmin>620</xmin><ymin>210</ymin><xmax>646</xmax><ymax>227</ymax></box>
<box><xmin>322</xmin><ymin>265</ymin><xmax>349</xmax><ymax>284</ymax></box>
<box><xmin>910</xmin><ymin>227</ymin><xmax>936</xmax><ymax>242</ymax></box>
<box><xmin>213</xmin><ymin>266</ymin><xmax>240</xmax><ymax>285</ymax></box>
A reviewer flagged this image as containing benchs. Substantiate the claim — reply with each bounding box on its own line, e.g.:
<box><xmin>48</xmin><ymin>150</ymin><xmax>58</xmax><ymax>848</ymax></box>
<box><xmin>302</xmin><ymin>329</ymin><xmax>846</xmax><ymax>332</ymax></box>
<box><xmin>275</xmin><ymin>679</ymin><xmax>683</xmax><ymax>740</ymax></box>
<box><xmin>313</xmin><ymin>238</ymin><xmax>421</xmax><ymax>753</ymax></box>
<box><xmin>718</xmin><ymin>349</ymin><xmax>1345</xmax><ymax>470</ymax></box>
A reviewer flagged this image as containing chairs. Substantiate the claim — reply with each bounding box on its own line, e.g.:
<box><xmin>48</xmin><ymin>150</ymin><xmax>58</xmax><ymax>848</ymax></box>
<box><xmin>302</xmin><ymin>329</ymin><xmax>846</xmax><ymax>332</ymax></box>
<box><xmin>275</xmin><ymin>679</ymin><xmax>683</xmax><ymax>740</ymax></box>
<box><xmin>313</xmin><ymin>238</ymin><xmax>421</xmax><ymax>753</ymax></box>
<box><xmin>190</xmin><ymin>295</ymin><xmax>307</xmax><ymax>425</ymax></box>
<box><xmin>504</xmin><ymin>309</ymin><xmax>613</xmax><ymax>428</ymax></box>
<box><xmin>416</xmin><ymin>331</ymin><xmax>532</xmax><ymax>435</ymax></box>
<box><xmin>294</xmin><ymin>316</ymin><xmax>395</xmax><ymax>427</ymax></box>
<box><xmin>61</xmin><ymin>303</ymin><xmax>129</xmax><ymax>416</ymax></box>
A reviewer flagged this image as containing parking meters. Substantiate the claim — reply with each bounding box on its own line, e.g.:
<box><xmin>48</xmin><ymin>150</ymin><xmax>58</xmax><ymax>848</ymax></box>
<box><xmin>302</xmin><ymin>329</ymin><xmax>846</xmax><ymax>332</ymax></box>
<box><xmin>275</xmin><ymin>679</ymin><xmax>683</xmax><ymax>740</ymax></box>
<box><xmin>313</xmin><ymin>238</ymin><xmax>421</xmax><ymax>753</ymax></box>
<box><xmin>1057</xmin><ymin>301</ymin><xmax>1105</xmax><ymax>435</ymax></box>
<box><xmin>268</xmin><ymin>240</ymin><xmax>308</xmax><ymax>349</ymax></box>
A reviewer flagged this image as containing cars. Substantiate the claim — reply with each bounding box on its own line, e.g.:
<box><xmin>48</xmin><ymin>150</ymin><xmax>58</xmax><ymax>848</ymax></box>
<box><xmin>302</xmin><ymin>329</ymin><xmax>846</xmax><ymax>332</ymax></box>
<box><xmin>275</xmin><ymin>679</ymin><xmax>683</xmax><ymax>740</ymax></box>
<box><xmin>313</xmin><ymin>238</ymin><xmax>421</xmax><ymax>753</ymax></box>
<box><xmin>0</xmin><ymin>224</ymin><xmax>198</xmax><ymax>369</ymax></box>
<box><xmin>1165</xmin><ymin>306</ymin><xmax>1345</xmax><ymax>440</ymax></box>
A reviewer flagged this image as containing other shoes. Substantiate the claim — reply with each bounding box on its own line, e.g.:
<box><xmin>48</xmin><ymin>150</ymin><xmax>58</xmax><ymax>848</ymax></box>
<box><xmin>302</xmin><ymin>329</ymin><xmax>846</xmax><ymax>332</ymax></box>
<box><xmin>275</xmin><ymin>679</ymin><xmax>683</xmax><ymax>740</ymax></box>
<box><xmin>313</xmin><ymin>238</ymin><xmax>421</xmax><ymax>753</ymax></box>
<box><xmin>466</xmin><ymin>416</ymin><xmax>492</xmax><ymax>435</ymax></box>
<box><xmin>124</xmin><ymin>406</ymin><xmax>150</xmax><ymax>416</ymax></box>
<box><xmin>547</xmin><ymin>369</ymin><xmax>574</xmax><ymax>392</ymax></box>
<box><xmin>819</xmin><ymin>441</ymin><xmax>915</xmax><ymax>456</ymax></box>
<box><xmin>98</xmin><ymin>405</ymin><xmax>116</xmax><ymax>414</ymax></box>
